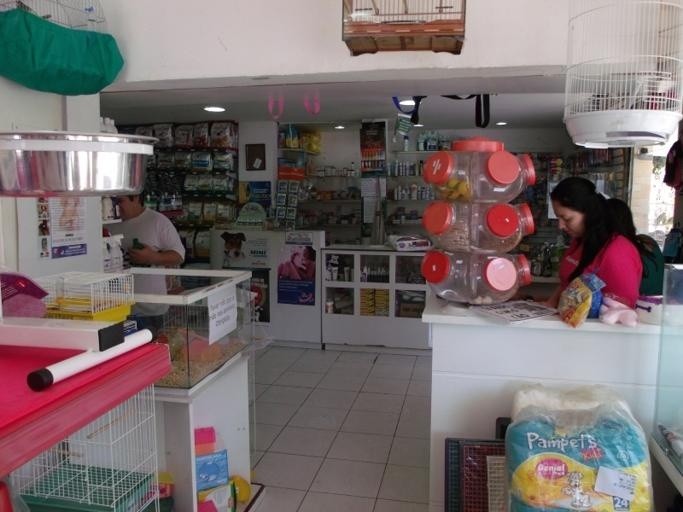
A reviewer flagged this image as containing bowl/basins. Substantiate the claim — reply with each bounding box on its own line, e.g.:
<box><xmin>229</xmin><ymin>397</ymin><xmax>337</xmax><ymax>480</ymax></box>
<box><xmin>0</xmin><ymin>131</ymin><xmax>159</xmax><ymax>197</ymax></box>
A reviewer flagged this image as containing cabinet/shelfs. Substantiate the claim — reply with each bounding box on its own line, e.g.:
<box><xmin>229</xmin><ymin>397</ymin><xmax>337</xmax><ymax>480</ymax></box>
<box><xmin>299</xmin><ymin>149</ymin><xmax>469</xmax><ymax>351</ymax></box>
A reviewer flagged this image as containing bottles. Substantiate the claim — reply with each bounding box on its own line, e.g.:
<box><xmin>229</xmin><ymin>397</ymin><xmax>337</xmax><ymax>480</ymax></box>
<box><xmin>389</xmin><ymin>160</ymin><xmax>435</xmax><ymax>202</ymax></box>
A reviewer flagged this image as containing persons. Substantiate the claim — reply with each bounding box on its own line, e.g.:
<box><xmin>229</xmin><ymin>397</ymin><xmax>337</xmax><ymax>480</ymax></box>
<box><xmin>595</xmin><ymin>197</ymin><xmax>664</xmax><ymax>297</ymax></box>
<box><xmin>542</xmin><ymin>176</ymin><xmax>644</xmax><ymax>319</ymax></box>
<box><xmin>101</xmin><ymin>187</ymin><xmax>187</xmax><ymax>336</ymax></box>
